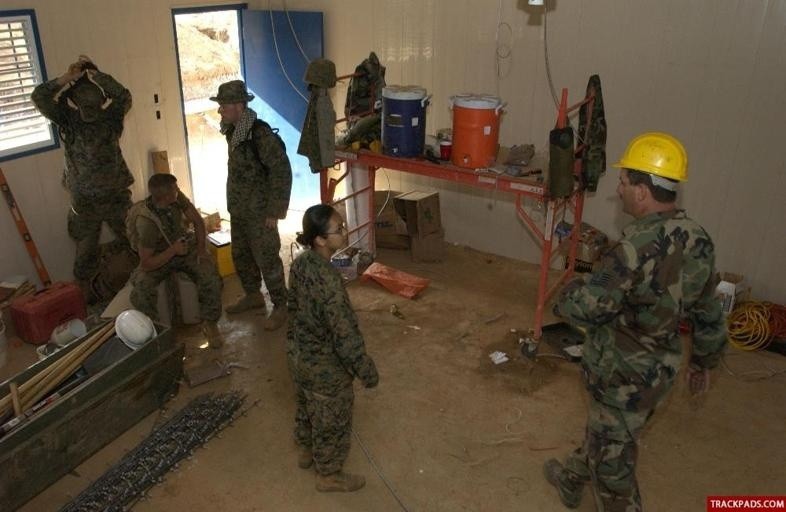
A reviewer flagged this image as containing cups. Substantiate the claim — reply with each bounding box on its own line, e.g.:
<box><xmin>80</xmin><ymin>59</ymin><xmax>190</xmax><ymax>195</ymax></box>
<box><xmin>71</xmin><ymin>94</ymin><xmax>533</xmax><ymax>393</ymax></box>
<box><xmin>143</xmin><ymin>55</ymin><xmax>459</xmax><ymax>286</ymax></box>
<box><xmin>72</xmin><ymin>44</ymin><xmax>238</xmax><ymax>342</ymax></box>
<box><xmin>440</xmin><ymin>142</ymin><xmax>451</xmax><ymax>161</ymax></box>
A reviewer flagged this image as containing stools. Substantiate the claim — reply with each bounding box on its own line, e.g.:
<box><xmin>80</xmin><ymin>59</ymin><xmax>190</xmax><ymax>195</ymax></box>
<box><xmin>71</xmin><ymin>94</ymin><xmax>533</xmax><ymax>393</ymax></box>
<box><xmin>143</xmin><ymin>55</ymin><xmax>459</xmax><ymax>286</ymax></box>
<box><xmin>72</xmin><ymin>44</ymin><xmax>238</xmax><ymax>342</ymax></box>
<box><xmin>153</xmin><ymin>274</ymin><xmax>200</xmax><ymax>326</ymax></box>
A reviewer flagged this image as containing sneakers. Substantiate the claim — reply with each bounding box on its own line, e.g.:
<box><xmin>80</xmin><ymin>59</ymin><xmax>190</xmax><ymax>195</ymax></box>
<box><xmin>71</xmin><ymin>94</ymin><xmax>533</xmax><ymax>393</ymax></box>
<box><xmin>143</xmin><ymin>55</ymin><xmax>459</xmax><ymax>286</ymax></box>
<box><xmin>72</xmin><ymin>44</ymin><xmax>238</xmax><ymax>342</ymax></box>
<box><xmin>224</xmin><ymin>292</ymin><xmax>266</xmax><ymax>314</ymax></box>
<box><xmin>543</xmin><ymin>458</ymin><xmax>582</xmax><ymax>508</ymax></box>
<box><xmin>200</xmin><ymin>319</ymin><xmax>226</xmax><ymax>348</ymax></box>
<box><xmin>263</xmin><ymin>304</ymin><xmax>289</xmax><ymax>332</ymax></box>
<box><xmin>315</xmin><ymin>467</ymin><xmax>366</xmax><ymax>492</ymax></box>
<box><xmin>297</xmin><ymin>449</ymin><xmax>314</xmax><ymax>468</ymax></box>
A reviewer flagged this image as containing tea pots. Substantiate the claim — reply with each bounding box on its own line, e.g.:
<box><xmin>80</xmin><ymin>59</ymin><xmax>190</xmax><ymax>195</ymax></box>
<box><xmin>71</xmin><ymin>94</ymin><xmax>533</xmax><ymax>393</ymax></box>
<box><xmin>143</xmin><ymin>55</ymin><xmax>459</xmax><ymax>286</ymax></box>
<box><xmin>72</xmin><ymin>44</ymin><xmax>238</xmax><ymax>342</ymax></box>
<box><xmin>357</xmin><ymin>251</ymin><xmax>377</xmax><ymax>274</ymax></box>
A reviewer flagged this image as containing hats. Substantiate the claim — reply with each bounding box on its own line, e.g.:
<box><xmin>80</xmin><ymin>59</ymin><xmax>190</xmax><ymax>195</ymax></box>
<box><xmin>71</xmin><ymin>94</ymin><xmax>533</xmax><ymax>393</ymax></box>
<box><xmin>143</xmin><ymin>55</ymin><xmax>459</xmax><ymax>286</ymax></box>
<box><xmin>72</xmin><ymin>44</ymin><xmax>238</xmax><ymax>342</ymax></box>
<box><xmin>209</xmin><ymin>80</ymin><xmax>255</xmax><ymax>103</ymax></box>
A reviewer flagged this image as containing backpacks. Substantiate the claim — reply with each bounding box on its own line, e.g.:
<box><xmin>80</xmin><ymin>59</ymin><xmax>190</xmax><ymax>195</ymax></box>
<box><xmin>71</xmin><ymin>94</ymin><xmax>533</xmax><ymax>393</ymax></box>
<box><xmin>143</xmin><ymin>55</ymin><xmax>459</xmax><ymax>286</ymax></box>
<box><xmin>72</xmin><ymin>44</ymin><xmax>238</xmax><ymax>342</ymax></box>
<box><xmin>124</xmin><ymin>199</ymin><xmax>146</xmax><ymax>253</ymax></box>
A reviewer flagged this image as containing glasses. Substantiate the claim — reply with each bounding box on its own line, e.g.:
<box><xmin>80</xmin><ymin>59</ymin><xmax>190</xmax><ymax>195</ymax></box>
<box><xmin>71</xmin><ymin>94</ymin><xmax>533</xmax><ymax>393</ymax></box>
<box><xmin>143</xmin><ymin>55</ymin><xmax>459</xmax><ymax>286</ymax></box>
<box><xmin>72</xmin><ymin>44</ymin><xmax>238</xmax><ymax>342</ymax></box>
<box><xmin>314</xmin><ymin>220</ymin><xmax>346</xmax><ymax>236</ymax></box>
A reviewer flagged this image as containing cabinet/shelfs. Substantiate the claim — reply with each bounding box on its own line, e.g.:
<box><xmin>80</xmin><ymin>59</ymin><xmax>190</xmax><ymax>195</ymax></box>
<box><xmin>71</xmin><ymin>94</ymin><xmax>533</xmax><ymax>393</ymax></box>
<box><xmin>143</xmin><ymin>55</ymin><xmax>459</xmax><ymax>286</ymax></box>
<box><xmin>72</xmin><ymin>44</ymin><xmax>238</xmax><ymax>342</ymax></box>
<box><xmin>307</xmin><ymin>72</ymin><xmax>594</xmax><ymax>342</ymax></box>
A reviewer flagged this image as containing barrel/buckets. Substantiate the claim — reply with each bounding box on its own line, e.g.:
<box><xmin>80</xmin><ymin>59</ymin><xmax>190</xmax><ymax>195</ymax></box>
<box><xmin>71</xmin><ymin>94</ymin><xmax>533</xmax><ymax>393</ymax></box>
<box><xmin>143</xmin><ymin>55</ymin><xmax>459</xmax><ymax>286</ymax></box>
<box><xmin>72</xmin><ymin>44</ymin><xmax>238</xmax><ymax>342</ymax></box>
<box><xmin>447</xmin><ymin>92</ymin><xmax>508</xmax><ymax>169</ymax></box>
<box><xmin>374</xmin><ymin>84</ymin><xmax>434</xmax><ymax>158</ymax></box>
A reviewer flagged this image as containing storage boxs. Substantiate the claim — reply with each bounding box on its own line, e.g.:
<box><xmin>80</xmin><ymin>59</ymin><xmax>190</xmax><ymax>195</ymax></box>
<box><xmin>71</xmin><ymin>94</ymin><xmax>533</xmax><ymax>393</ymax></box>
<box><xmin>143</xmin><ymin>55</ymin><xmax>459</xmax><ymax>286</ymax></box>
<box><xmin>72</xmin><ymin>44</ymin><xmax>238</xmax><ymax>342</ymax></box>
<box><xmin>713</xmin><ymin>270</ymin><xmax>747</xmax><ymax>320</ymax></box>
<box><xmin>393</xmin><ymin>191</ymin><xmax>441</xmax><ymax>233</ymax></box>
<box><xmin>558</xmin><ymin>237</ymin><xmax>602</xmax><ymax>263</ymax></box>
<box><xmin>10</xmin><ymin>283</ymin><xmax>85</xmax><ymax>343</ymax></box>
<box><xmin>373</xmin><ymin>190</ymin><xmax>403</xmax><ymax>225</ymax></box>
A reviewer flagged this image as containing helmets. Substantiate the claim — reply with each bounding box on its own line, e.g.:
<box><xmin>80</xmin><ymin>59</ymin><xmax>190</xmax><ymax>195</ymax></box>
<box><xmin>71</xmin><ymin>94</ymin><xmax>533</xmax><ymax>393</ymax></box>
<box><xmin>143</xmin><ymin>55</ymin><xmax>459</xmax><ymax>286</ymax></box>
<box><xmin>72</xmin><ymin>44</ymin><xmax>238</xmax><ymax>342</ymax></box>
<box><xmin>302</xmin><ymin>58</ymin><xmax>336</xmax><ymax>90</ymax></box>
<box><xmin>35</xmin><ymin>343</ymin><xmax>65</xmax><ymax>361</ymax></box>
<box><xmin>115</xmin><ymin>309</ymin><xmax>157</xmax><ymax>351</ymax></box>
<box><xmin>611</xmin><ymin>132</ymin><xmax>690</xmax><ymax>193</ymax></box>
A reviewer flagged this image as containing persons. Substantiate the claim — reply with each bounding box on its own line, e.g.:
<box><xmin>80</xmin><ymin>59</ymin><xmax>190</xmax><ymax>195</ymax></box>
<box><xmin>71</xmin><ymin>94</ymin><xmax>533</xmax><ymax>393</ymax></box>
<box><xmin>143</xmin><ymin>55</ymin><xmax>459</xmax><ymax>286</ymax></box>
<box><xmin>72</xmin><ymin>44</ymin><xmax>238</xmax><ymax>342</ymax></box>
<box><xmin>283</xmin><ymin>205</ymin><xmax>380</xmax><ymax>493</ymax></box>
<box><xmin>542</xmin><ymin>131</ymin><xmax>727</xmax><ymax>512</ymax></box>
<box><xmin>30</xmin><ymin>53</ymin><xmax>138</xmax><ymax>304</ymax></box>
<box><xmin>209</xmin><ymin>80</ymin><xmax>292</xmax><ymax>330</ymax></box>
<box><xmin>125</xmin><ymin>174</ymin><xmax>224</xmax><ymax>348</ymax></box>
